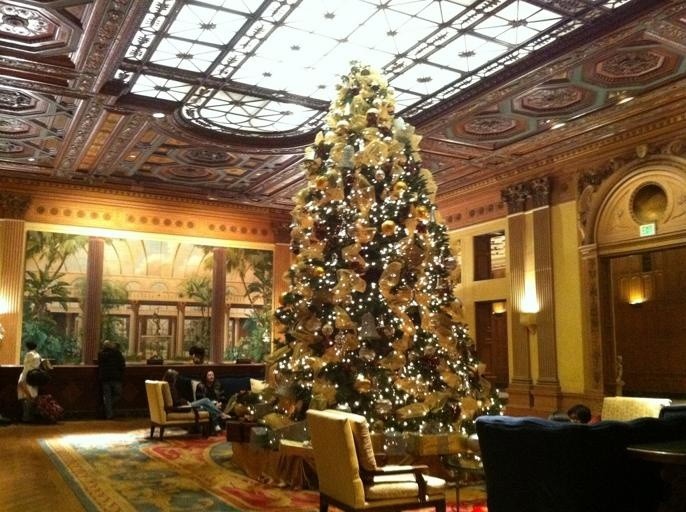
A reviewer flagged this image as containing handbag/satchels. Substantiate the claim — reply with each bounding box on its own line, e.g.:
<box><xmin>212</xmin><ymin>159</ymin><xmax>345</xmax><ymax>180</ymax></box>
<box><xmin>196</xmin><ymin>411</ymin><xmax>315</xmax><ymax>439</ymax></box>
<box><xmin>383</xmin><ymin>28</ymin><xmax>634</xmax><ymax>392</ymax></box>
<box><xmin>27</xmin><ymin>368</ymin><xmax>49</xmax><ymax>385</ymax></box>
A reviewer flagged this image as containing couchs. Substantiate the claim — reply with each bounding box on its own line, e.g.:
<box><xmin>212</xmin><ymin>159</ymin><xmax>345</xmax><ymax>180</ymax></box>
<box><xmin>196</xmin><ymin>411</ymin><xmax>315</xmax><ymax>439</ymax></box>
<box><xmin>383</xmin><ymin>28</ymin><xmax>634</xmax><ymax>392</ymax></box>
<box><xmin>475</xmin><ymin>405</ymin><xmax>686</xmax><ymax>512</ymax></box>
<box><xmin>178</xmin><ymin>372</ymin><xmax>253</xmax><ymax>430</ymax></box>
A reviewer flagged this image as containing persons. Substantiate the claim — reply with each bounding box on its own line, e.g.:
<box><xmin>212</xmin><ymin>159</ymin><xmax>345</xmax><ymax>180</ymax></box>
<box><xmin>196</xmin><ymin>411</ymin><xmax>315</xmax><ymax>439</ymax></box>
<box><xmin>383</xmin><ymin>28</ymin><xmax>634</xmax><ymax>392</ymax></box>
<box><xmin>98</xmin><ymin>339</ymin><xmax>125</xmax><ymax>419</ymax></box>
<box><xmin>16</xmin><ymin>342</ymin><xmax>42</xmax><ymax>421</ymax></box>
<box><xmin>162</xmin><ymin>369</ymin><xmax>231</xmax><ymax>431</ymax></box>
<box><xmin>568</xmin><ymin>404</ymin><xmax>592</xmax><ymax>423</ymax></box>
<box><xmin>196</xmin><ymin>369</ymin><xmax>227</xmax><ymax>436</ymax></box>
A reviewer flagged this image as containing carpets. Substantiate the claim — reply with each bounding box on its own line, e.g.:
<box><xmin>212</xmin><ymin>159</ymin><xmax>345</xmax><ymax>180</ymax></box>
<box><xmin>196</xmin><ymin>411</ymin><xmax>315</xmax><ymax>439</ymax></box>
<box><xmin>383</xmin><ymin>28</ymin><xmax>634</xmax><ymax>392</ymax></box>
<box><xmin>35</xmin><ymin>431</ymin><xmax>489</xmax><ymax>512</ymax></box>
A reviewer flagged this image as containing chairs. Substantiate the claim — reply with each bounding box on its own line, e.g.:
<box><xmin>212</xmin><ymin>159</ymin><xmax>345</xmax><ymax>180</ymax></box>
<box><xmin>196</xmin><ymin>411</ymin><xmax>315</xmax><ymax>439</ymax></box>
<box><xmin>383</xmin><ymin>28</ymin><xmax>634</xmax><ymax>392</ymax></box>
<box><xmin>307</xmin><ymin>407</ymin><xmax>446</xmax><ymax>512</ymax></box>
<box><xmin>145</xmin><ymin>380</ymin><xmax>210</xmax><ymax>440</ymax></box>
<box><xmin>601</xmin><ymin>395</ymin><xmax>672</xmax><ymax>421</ymax></box>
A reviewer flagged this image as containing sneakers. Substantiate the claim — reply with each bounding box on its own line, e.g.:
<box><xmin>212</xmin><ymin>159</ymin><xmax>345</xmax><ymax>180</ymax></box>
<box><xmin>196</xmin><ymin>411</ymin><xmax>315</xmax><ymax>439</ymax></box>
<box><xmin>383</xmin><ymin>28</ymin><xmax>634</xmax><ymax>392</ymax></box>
<box><xmin>214</xmin><ymin>425</ymin><xmax>221</xmax><ymax>431</ymax></box>
<box><xmin>221</xmin><ymin>414</ymin><xmax>232</xmax><ymax>419</ymax></box>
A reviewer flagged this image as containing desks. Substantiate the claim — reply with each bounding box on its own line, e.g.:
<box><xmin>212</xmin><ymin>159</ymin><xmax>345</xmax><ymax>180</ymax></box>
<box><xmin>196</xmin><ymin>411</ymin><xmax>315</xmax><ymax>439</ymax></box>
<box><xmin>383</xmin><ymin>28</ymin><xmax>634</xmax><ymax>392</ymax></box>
<box><xmin>625</xmin><ymin>439</ymin><xmax>686</xmax><ymax>512</ymax></box>
<box><xmin>445</xmin><ymin>454</ymin><xmax>484</xmax><ymax>512</ymax></box>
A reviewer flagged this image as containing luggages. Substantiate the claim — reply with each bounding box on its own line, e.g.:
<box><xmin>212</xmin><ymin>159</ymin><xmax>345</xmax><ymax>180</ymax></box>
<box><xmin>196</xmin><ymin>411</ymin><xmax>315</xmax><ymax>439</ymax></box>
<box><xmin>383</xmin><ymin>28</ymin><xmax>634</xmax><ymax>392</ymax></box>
<box><xmin>19</xmin><ymin>382</ymin><xmax>64</xmax><ymax>422</ymax></box>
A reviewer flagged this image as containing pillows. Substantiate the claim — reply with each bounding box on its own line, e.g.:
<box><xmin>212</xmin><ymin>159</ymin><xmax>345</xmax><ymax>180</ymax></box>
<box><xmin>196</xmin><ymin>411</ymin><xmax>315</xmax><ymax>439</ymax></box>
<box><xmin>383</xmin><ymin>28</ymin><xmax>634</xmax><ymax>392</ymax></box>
<box><xmin>157</xmin><ymin>381</ymin><xmax>173</xmax><ymax>407</ymax></box>
<box><xmin>322</xmin><ymin>409</ymin><xmax>376</xmax><ymax>473</ymax></box>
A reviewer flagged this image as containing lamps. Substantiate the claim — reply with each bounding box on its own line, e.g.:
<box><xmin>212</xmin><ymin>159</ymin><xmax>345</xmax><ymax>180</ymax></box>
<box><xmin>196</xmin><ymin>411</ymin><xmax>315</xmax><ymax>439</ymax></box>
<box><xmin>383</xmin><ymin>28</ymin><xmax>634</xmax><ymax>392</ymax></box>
<box><xmin>492</xmin><ymin>303</ymin><xmax>506</xmax><ymax>317</ymax></box>
